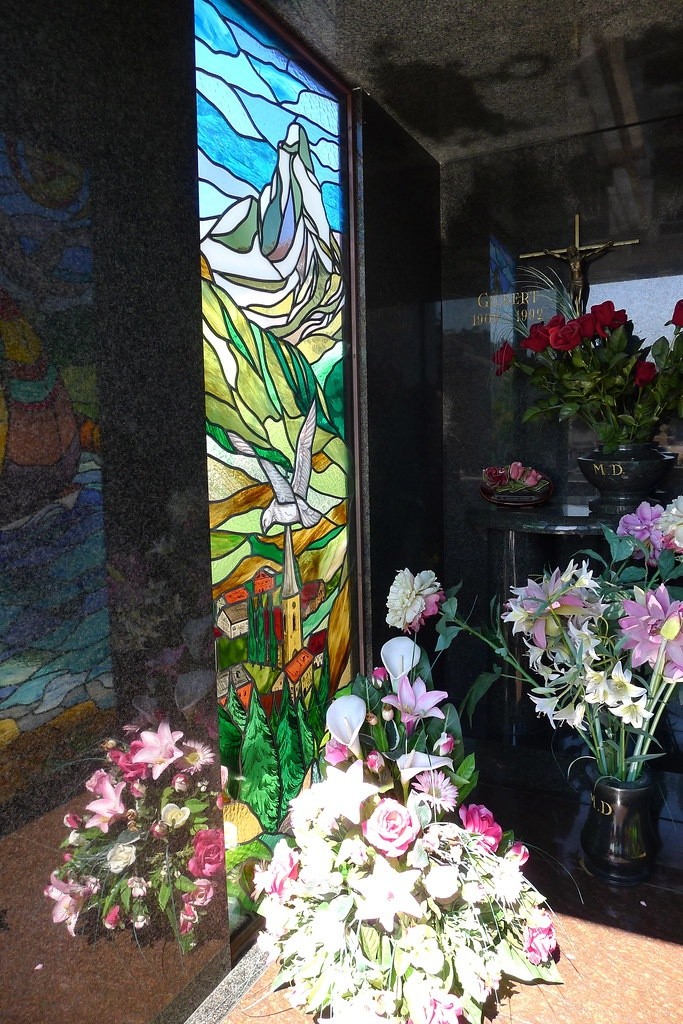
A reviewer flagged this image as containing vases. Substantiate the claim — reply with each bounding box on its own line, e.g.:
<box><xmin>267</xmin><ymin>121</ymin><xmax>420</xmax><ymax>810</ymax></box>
<box><xmin>575</xmin><ymin>441</ymin><xmax>679</xmax><ymax>514</ymax></box>
<box><xmin>580</xmin><ymin>760</ymin><xmax>660</xmax><ymax>886</ymax></box>
<box><xmin>479</xmin><ymin>471</ymin><xmax>553</xmax><ymax>509</ymax></box>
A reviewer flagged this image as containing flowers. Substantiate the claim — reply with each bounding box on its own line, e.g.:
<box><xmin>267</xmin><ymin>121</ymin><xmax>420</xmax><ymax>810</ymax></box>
<box><xmin>483</xmin><ymin>462</ymin><xmax>550</xmax><ymax>493</ymax></box>
<box><xmin>43</xmin><ymin>643</ymin><xmax>228</xmax><ymax>973</ymax></box>
<box><xmin>481</xmin><ymin>265</ymin><xmax>683</xmax><ymax>455</ymax></box>
<box><xmin>386</xmin><ymin>497</ymin><xmax>683</xmax><ymax>796</ymax></box>
<box><xmin>254</xmin><ymin>595</ymin><xmax>584</xmax><ymax>1024</ymax></box>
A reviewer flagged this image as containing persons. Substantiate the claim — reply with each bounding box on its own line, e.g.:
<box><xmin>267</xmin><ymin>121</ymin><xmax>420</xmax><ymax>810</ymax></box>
<box><xmin>544</xmin><ymin>240</ymin><xmax>614</xmax><ymax>315</ymax></box>
